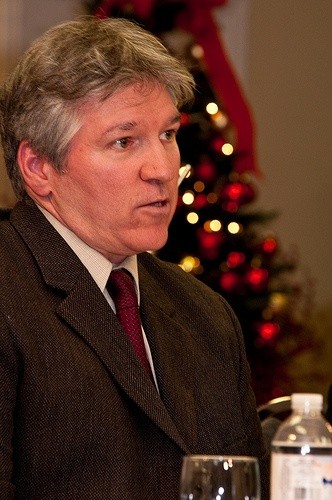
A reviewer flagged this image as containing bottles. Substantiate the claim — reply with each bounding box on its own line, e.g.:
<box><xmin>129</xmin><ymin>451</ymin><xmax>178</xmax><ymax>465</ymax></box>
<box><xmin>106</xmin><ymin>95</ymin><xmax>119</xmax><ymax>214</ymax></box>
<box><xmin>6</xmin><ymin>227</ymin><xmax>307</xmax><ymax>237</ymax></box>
<box><xmin>270</xmin><ymin>392</ymin><xmax>332</xmax><ymax>500</ymax></box>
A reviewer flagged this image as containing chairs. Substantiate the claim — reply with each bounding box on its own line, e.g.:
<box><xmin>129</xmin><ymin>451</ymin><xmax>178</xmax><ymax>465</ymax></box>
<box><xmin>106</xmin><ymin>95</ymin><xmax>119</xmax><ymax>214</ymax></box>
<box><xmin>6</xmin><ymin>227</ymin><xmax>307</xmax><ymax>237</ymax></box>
<box><xmin>256</xmin><ymin>395</ymin><xmax>328</xmax><ymax>460</ymax></box>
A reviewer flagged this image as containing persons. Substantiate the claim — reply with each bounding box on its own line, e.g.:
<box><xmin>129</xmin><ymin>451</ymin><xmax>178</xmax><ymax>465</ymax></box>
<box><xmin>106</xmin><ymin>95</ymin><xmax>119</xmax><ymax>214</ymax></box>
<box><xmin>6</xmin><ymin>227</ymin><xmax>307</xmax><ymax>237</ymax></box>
<box><xmin>0</xmin><ymin>17</ymin><xmax>270</xmax><ymax>500</ymax></box>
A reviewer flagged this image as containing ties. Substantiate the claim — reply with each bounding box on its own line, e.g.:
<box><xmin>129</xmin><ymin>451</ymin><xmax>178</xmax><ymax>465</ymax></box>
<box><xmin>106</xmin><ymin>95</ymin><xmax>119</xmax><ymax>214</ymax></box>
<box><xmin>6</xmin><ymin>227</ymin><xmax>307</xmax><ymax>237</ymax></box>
<box><xmin>106</xmin><ymin>268</ymin><xmax>156</xmax><ymax>394</ymax></box>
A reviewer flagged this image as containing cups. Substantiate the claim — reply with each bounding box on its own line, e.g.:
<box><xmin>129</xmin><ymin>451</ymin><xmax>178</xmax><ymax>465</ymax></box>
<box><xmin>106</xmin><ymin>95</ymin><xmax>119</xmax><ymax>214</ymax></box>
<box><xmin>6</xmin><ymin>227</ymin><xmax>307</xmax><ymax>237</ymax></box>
<box><xmin>179</xmin><ymin>455</ymin><xmax>260</xmax><ymax>500</ymax></box>
